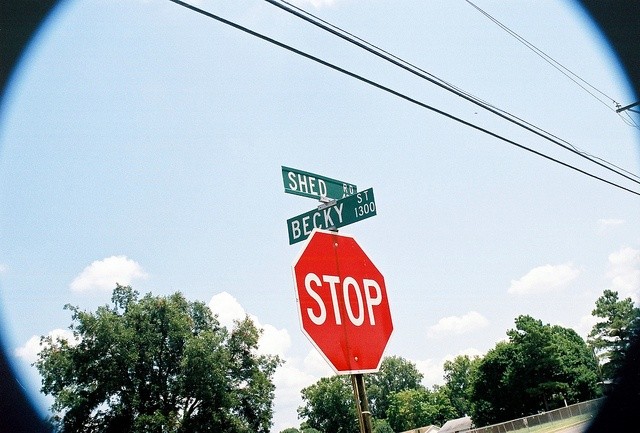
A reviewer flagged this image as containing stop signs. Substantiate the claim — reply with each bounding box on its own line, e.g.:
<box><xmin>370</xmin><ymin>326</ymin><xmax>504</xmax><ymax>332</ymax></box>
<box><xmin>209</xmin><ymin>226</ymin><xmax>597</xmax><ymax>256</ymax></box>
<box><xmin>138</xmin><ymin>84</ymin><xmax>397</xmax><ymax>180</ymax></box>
<box><xmin>291</xmin><ymin>228</ymin><xmax>393</xmax><ymax>375</ymax></box>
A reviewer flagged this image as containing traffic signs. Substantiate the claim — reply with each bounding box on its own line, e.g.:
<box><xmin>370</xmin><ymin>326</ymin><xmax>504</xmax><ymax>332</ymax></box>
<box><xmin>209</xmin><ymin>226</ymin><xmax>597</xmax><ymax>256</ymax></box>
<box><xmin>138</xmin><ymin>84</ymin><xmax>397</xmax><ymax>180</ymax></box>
<box><xmin>287</xmin><ymin>188</ymin><xmax>376</xmax><ymax>245</ymax></box>
<box><xmin>282</xmin><ymin>166</ymin><xmax>357</xmax><ymax>199</ymax></box>
